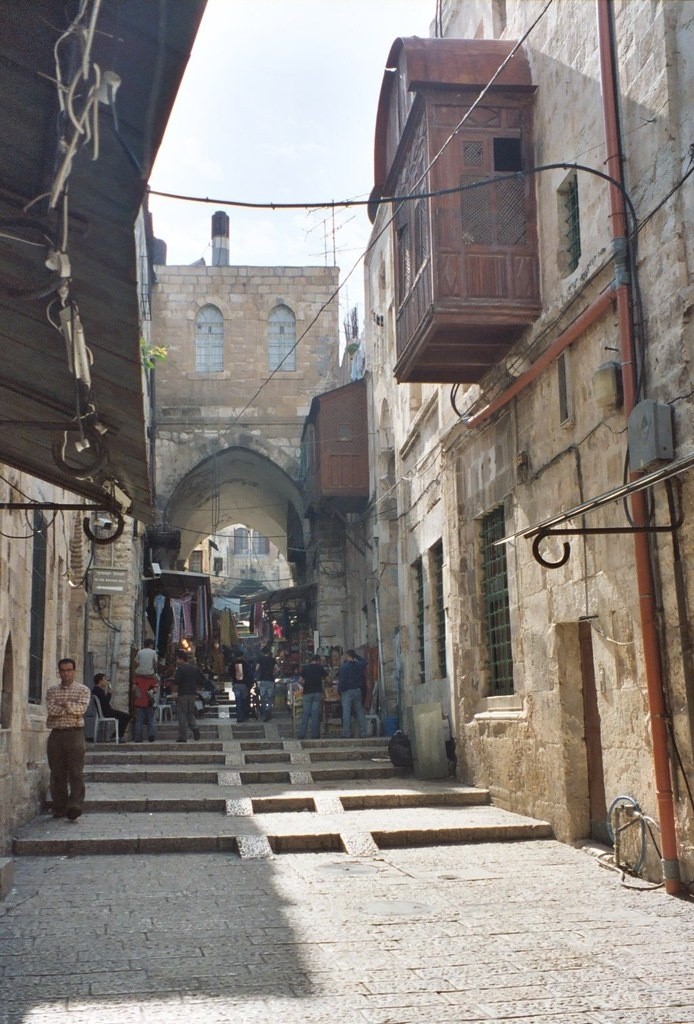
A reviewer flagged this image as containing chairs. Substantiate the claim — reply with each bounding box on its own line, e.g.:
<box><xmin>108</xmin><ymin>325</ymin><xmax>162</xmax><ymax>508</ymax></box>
<box><xmin>91</xmin><ymin>697</ymin><xmax>119</xmax><ymax>742</ymax></box>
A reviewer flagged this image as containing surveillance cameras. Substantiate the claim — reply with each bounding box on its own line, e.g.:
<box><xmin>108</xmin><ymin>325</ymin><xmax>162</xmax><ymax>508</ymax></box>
<box><xmin>150</xmin><ymin>562</ymin><xmax>162</xmax><ymax>579</ymax></box>
<box><xmin>93</xmin><ymin>518</ymin><xmax>112</xmax><ymax>530</ymax></box>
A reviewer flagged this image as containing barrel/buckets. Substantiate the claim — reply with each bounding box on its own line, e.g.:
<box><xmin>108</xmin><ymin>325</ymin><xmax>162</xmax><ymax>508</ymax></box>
<box><xmin>384</xmin><ymin>716</ymin><xmax>399</xmax><ymax>735</ymax></box>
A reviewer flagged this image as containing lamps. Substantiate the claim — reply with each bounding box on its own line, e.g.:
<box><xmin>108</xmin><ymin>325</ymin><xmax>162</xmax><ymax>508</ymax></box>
<box><xmin>593</xmin><ymin>361</ymin><xmax>623</xmax><ymax>413</ymax></box>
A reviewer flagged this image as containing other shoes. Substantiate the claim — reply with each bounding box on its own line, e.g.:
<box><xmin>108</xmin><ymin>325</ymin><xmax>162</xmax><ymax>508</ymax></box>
<box><xmin>135</xmin><ymin>739</ymin><xmax>143</xmax><ymax>743</ymax></box>
<box><xmin>193</xmin><ymin>727</ymin><xmax>200</xmax><ymax>740</ymax></box>
<box><xmin>110</xmin><ymin>735</ymin><xmax>126</xmax><ymax>743</ymax></box>
<box><xmin>54</xmin><ymin>808</ymin><xmax>82</xmax><ymax>820</ymax></box>
<box><xmin>176</xmin><ymin>738</ymin><xmax>187</xmax><ymax>742</ymax></box>
<box><xmin>237</xmin><ymin>716</ymin><xmax>250</xmax><ymax>722</ymax></box>
<box><xmin>297</xmin><ymin>736</ymin><xmax>304</xmax><ymax>739</ymax></box>
<box><xmin>310</xmin><ymin>736</ymin><xmax>320</xmax><ymax>739</ymax></box>
<box><xmin>264</xmin><ymin>712</ymin><xmax>272</xmax><ymax>722</ymax></box>
<box><xmin>149</xmin><ymin>736</ymin><xmax>156</xmax><ymax>742</ymax></box>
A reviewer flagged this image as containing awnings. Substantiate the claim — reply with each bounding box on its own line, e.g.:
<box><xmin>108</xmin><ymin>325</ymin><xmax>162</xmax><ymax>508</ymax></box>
<box><xmin>154</xmin><ymin>570</ymin><xmax>212</xmax><ymax>608</ymax></box>
<box><xmin>267</xmin><ymin>583</ymin><xmax>318</xmax><ymax>603</ymax></box>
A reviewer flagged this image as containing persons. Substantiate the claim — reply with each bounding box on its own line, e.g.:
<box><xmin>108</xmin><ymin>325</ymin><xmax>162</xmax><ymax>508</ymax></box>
<box><xmin>131</xmin><ymin>639</ymin><xmax>160</xmax><ymax>704</ymax></box>
<box><xmin>46</xmin><ymin>659</ymin><xmax>90</xmax><ymax>821</ymax></box>
<box><xmin>171</xmin><ymin>650</ymin><xmax>201</xmax><ymax>743</ymax></box>
<box><xmin>255</xmin><ymin>646</ymin><xmax>279</xmax><ymax>721</ymax></box>
<box><xmin>134</xmin><ymin>676</ymin><xmax>158</xmax><ymax>743</ymax></box>
<box><xmin>93</xmin><ymin>673</ymin><xmax>131</xmax><ymax>744</ymax></box>
<box><xmin>298</xmin><ymin>654</ymin><xmax>328</xmax><ymax>740</ymax></box>
<box><xmin>336</xmin><ymin>650</ymin><xmax>369</xmax><ymax>738</ymax></box>
<box><xmin>227</xmin><ymin>651</ymin><xmax>256</xmax><ymax>723</ymax></box>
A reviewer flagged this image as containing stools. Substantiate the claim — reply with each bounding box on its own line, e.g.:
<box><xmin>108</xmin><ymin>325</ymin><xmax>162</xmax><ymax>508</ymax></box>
<box><xmin>156</xmin><ymin>703</ymin><xmax>173</xmax><ymax>724</ymax></box>
<box><xmin>365</xmin><ymin>715</ymin><xmax>379</xmax><ymax>737</ymax></box>
